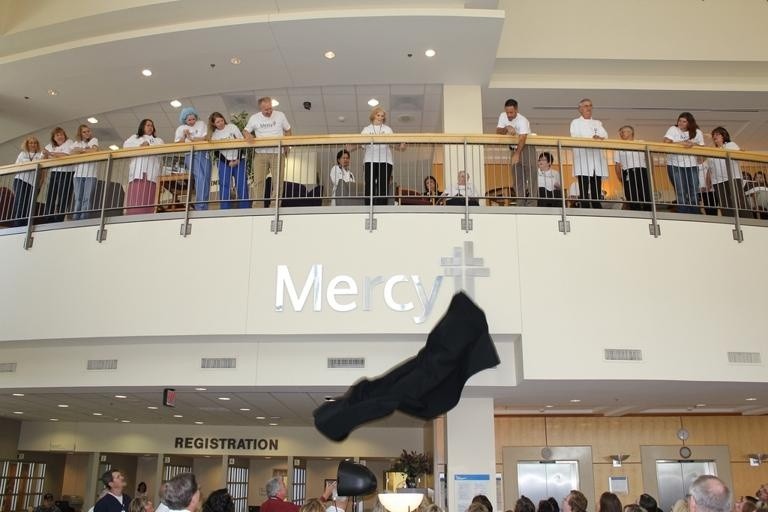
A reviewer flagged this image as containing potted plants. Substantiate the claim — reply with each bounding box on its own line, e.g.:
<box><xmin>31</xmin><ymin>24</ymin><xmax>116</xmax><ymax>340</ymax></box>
<box><xmin>386</xmin><ymin>448</ymin><xmax>432</xmax><ymax>489</ymax></box>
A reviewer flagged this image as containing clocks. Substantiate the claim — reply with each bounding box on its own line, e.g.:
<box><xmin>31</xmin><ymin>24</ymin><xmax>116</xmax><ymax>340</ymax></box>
<box><xmin>678</xmin><ymin>428</ymin><xmax>689</xmax><ymax>440</ymax></box>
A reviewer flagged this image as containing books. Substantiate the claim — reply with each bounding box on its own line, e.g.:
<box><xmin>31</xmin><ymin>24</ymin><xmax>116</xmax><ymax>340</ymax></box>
<box><xmin>228</xmin><ymin>468</ymin><xmax>247</xmax><ymax>510</ymax></box>
<box><xmin>293</xmin><ymin>470</ymin><xmax>306</xmax><ymax>506</ymax></box>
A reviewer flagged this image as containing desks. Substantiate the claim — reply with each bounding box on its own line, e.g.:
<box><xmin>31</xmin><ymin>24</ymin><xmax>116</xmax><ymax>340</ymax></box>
<box><xmin>157</xmin><ymin>175</ymin><xmax>194</xmax><ymax>212</ymax></box>
<box><xmin>485</xmin><ymin>187</ymin><xmax>514</xmax><ymax>206</ymax></box>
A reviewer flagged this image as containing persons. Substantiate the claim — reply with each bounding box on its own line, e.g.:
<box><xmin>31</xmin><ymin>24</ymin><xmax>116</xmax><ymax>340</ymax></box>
<box><xmin>11</xmin><ymin>136</ymin><xmax>44</xmax><ymax>227</ymax></box>
<box><xmin>242</xmin><ymin>96</ymin><xmax>292</xmax><ymax>208</ymax></box>
<box><xmin>424</xmin><ymin>176</ymin><xmax>448</xmax><ymax>201</ymax></box>
<box><xmin>495</xmin><ymin>99</ymin><xmax>768</xmax><ymax>220</ymax></box>
<box><xmin>569</xmin><ymin>180</ymin><xmax>580</xmax><ymax>207</ymax></box>
<box><xmin>360</xmin><ymin>106</ymin><xmax>405</xmax><ymax>205</ymax></box>
<box><xmin>36</xmin><ymin>470</ymin><xmax>234</xmax><ymax>512</ymax></box>
<box><xmin>174</xmin><ymin>108</ymin><xmax>210</xmax><ymax>211</ymax></box>
<box><xmin>330</xmin><ymin>149</ymin><xmax>356</xmax><ymax>207</ymax></box>
<box><xmin>446</xmin><ymin>170</ymin><xmax>480</xmax><ymax>201</ymax></box>
<box><xmin>69</xmin><ymin>124</ymin><xmax>100</xmax><ymax>221</ymax></box>
<box><xmin>209</xmin><ymin>111</ymin><xmax>250</xmax><ymax>210</ymax></box>
<box><xmin>259</xmin><ymin>477</ymin><xmax>349</xmax><ymax>512</ymax></box>
<box><xmin>122</xmin><ymin>118</ymin><xmax>165</xmax><ymax>215</ymax></box>
<box><xmin>425</xmin><ymin>475</ymin><xmax>768</xmax><ymax>511</ymax></box>
<box><xmin>39</xmin><ymin>127</ymin><xmax>74</xmax><ymax>222</ymax></box>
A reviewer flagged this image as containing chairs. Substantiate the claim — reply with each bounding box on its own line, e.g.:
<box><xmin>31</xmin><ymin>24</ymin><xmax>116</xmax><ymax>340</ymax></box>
<box><xmin>0</xmin><ymin>186</ymin><xmax>14</xmax><ymax>226</ymax></box>
<box><xmin>265</xmin><ymin>177</ymin><xmax>324</xmax><ymax>206</ymax></box>
<box><xmin>397</xmin><ymin>188</ymin><xmax>431</xmax><ymax>203</ymax></box>
<box><xmin>92</xmin><ymin>181</ymin><xmax>125</xmax><ymax>217</ymax></box>
